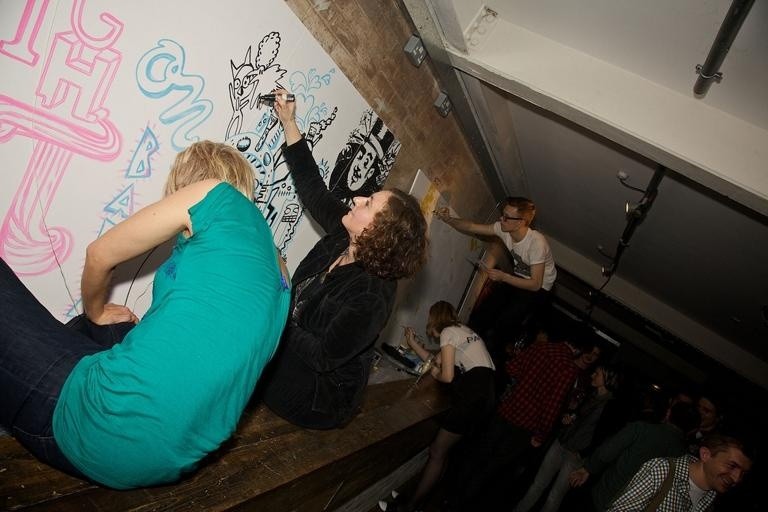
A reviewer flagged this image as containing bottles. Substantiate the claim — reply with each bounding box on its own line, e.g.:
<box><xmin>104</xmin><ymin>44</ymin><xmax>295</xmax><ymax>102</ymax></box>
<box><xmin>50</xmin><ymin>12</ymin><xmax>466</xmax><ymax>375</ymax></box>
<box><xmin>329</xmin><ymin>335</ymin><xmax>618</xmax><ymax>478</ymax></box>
<box><xmin>412</xmin><ymin>351</ymin><xmax>438</xmax><ymax>387</ymax></box>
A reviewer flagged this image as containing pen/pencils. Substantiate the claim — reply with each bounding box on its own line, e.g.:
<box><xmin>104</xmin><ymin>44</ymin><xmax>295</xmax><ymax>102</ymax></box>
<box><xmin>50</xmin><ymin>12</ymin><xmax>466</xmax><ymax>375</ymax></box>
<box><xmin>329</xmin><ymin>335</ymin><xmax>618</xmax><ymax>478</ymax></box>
<box><xmin>479</xmin><ymin>260</ymin><xmax>488</xmax><ymax>270</ymax></box>
<box><xmin>259</xmin><ymin>95</ymin><xmax>297</xmax><ymax>103</ymax></box>
<box><xmin>399</xmin><ymin>325</ymin><xmax>425</xmax><ymax>339</ymax></box>
<box><xmin>432</xmin><ymin>210</ymin><xmax>450</xmax><ymax>213</ymax></box>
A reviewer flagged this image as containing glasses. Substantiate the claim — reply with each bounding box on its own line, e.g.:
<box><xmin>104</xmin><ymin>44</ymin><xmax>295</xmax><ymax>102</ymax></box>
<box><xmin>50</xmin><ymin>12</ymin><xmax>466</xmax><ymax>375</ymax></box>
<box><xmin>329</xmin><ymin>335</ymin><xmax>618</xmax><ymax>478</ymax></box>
<box><xmin>502</xmin><ymin>212</ymin><xmax>524</xmax><ymax>224</ymax></box>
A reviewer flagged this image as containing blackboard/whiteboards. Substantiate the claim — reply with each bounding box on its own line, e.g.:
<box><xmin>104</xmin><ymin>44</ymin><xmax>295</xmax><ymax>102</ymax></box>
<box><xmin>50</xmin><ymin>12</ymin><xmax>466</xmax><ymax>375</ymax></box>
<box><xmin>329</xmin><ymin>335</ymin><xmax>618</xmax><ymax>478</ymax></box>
<box><xmin>1</xmin><ymin>0</ymin><xmax>402</xmax><ymax>324</ymax></box>
<box><xmin>374</xmin><ymin>169</ymin><xmax>485</xmax><ymax>351</ymax></box>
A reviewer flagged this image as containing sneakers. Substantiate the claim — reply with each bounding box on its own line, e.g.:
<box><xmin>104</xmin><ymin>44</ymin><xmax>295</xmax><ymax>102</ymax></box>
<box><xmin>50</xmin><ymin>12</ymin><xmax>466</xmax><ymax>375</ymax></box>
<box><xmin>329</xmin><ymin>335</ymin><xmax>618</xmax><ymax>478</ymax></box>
<box><xmin>378</xmin><ymin>490</ymin><xmax>409</xmax><ymax>512</ymax></box>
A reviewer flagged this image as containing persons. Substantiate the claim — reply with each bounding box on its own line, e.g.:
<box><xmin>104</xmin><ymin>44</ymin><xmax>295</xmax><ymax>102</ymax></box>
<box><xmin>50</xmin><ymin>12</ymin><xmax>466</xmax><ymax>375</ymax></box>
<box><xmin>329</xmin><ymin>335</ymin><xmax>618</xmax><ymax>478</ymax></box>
<box><xmin>434</xmin><ymin>197</ymin><xmax>559</xmax><ymax>359</ymax></box>
<box><xmin>514</xmin><ymin>329</ymin><xmax>768</xmax><ymax>512</ymax></box>
<box><xmin>258</xmin><ymin>89</ymin><xmax>432</xmax><ymax>430</ymax></box>
<box><xmin>0</xmin><ymin>140</ymin><xmax>292</xmax><ymax>490</ymax></box>
<box><xmin>379</xmin><ymin>299</ymin><xmax>497</xmax><ymax>512</ymax></box>
